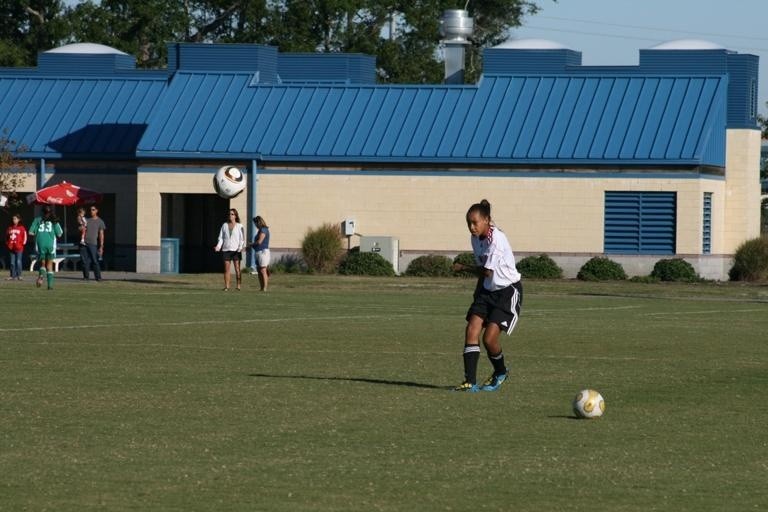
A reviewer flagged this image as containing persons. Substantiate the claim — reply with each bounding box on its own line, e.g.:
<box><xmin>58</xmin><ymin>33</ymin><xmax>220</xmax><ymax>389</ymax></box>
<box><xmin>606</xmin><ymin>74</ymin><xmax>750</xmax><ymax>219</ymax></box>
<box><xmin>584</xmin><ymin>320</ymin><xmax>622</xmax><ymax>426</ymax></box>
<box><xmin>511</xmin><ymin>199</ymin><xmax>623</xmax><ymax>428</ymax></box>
<box><xmin>4</xmin><ymin>211</ymin><xmax>28</xmax><ymax>282</ymax></box>
<box><xmin>28</xmin><ymin>205</ymin><xmax>64</xmax><ymax>290</ymax></box>
<box><xmin>73</xmin><ymin>204</ymin><xmax>106</xmax><ymax>282</ymax></box>
<box><xmin>451</xmin><ymin>197</ymin><xmax>523</xmax><ymax>394</ymax></box>
<box><xmin>211</xmin><ymin>208</ymin><xmax>246</xmax><ymax>292</ymax></box>
<box><xmin>74</xmin><ymin>208</ymin><xmax>88</xmax><ymax>247</ymax></box>
<box><xmin>247</xmin><ymin>214</ymin><xmax>270</xmax><ymax>293</ymax></box>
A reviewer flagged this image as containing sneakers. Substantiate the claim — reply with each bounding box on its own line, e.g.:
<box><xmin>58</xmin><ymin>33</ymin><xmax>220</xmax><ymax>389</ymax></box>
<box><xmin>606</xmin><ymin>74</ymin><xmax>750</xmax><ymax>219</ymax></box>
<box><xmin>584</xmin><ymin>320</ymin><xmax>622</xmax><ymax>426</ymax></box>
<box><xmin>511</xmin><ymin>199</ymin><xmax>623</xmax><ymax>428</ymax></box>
<box><xmin>5</xmin><ymin>274</ymin><xmax>103</xmax><ymax>291</ymax></box>
<box><xmin>453</xmin><ymin>380</ymin><xmax>479</xmax><ymax>394</ymax></box>
<box><xmin>480</xmin><ymin>366</ymin><xmax>510</xmax><ymax>392</ymax></box>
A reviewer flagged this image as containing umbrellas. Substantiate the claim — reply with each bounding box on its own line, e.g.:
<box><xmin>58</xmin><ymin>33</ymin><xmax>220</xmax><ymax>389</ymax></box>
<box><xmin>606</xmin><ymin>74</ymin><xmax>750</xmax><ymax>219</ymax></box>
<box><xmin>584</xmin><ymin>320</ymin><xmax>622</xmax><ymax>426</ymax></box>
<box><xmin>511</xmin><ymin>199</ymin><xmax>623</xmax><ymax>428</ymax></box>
<box><xmin>25</xmin><ymin>180</ymin><xmax>104</xmax><ymax>244</ymax></box>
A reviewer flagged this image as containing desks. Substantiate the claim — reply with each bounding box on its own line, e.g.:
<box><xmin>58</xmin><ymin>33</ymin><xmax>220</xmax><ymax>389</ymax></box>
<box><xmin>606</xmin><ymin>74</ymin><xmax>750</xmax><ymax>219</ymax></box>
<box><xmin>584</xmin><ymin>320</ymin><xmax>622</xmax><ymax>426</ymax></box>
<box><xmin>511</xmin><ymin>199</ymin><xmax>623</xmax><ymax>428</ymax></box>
<box><xmin>57</xmin><ymin>243</ymin><xmax>80</xmax><ymax>255</ymax></box>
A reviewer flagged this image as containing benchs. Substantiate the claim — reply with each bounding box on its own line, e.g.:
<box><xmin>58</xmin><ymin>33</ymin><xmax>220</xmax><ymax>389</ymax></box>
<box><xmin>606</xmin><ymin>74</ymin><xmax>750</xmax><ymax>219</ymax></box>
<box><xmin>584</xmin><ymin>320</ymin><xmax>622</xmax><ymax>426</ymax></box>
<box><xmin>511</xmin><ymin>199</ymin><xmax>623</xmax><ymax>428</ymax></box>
<box><xmin>29</xmin><ymin>254</ymin><xmax>105</xmax><ymax>275</ymax></box>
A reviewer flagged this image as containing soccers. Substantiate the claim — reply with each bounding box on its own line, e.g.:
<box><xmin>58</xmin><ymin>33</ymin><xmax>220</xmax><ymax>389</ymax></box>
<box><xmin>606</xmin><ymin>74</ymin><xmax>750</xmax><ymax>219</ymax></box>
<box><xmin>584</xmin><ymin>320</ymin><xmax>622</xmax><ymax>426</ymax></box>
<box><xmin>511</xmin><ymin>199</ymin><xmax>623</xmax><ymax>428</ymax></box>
<box><xmin>213</xmin><ymin>164</ymin><xmax>246</xmax><ymax>200</ymax></box>
<box><xmin>574</xmin><ymin>389</ymin><xmax>606</xmax><ymax>419</ymax></box>
